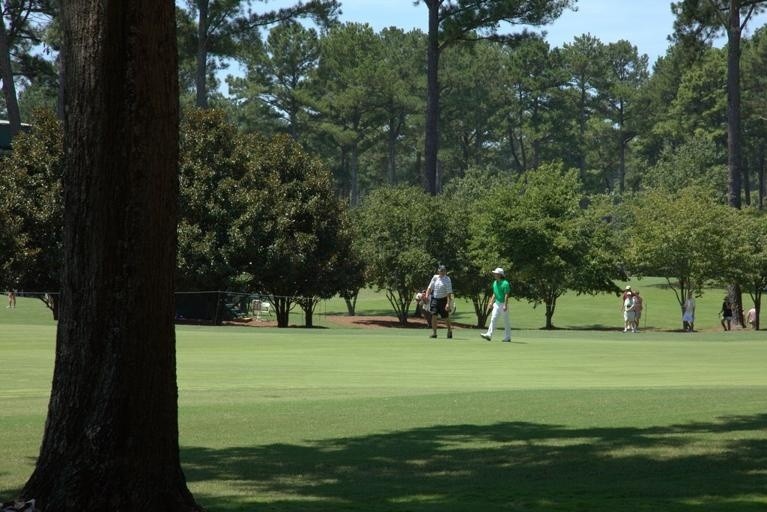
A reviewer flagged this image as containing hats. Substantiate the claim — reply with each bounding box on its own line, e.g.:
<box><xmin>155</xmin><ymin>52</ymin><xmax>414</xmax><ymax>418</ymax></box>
<box><xmin>492</xmin><ymin>267</ymin><xmax>505</xmax><ymax>275</ymax></box>
<box><xmin>627</xmin><ymin>292</ymin><xmax>631</xmax><ymax>296</ymax></box>
<box><xmin>438</xmin><ymin>265</ymin><xmax>446</xmax><ymax>271</ymax></box>
<box><xmin>625</xmin><ymin>286</ymin><xmax>631</xmax><ymax>290</ymax></box>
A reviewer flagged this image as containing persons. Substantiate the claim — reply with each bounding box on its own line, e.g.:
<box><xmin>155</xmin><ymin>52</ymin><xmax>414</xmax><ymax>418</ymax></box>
<box><xmin>741</xmin><ymin>306</ymin><xmax>746</xmax><ymax>327</ymax></box>
<box><xmin>622</xmin><ymin>291</ymin><xmax>637</xmax><ymax>334</ymax></box>
<box><xmin>479</xmin><ymin>266</ymin><xmax>513</xmax><ymax>343</ymax></box>
<box><xmin>620</xmin><ymin>285</ymin><xmax>637</xmax><ymax>330</ymax></box>
<box><xmin>681</xmin><ymin>290</ymin><xmax>696</xmax><ymax>333</ymax></box>
<box><xmin>632</xmin><ymin>289</ymin><xmax>643</xmax><ymax>332</ymax></box>
<box><xmin>745</xmin><ymin>304</ymin><xmax>758</xmax><ymax>329</ymax></box>
<box><xmin>718</xmin><ymin>296</ymin><xmax>733</xmax><ymax>332</ymax></box>
<box><xmin>423</xmin><ymin>263</ymin><xmax>454</xmax><ymax>339</ymax></box>
<box><xmin>417</xmin><ymin>289</ymin><xmax>432</xmax><ymax>328</ymax></box>
<box><xmin>5</xmin><ymin>289</ymin><xmax>17</xmax><ymax>309</ymax></box>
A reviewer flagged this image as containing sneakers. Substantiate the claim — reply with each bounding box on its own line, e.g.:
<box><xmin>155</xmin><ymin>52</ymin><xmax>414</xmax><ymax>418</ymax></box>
<box><xmin>623</xmin><ymin>325</ymin><xmax>639</xmax><ymax>333</ymax></box>
<box><xmin>429</xmin><ymin>334</ymin><xmax>437</xmax><ymax>338</ymax></box>
<box><xmin>446</xmin><ymin>331</ymin><xmax>453</xmax><ymax>338</ymax></box>
<box><xmin>502</xmin><ymin>338</ymin><xmax>512</xmax><ymax>342</ymax></box>
<box><xmin>480</xmin><ymin>333</ymin><xmax>491</xmax><ymax>341</ymax></box>
